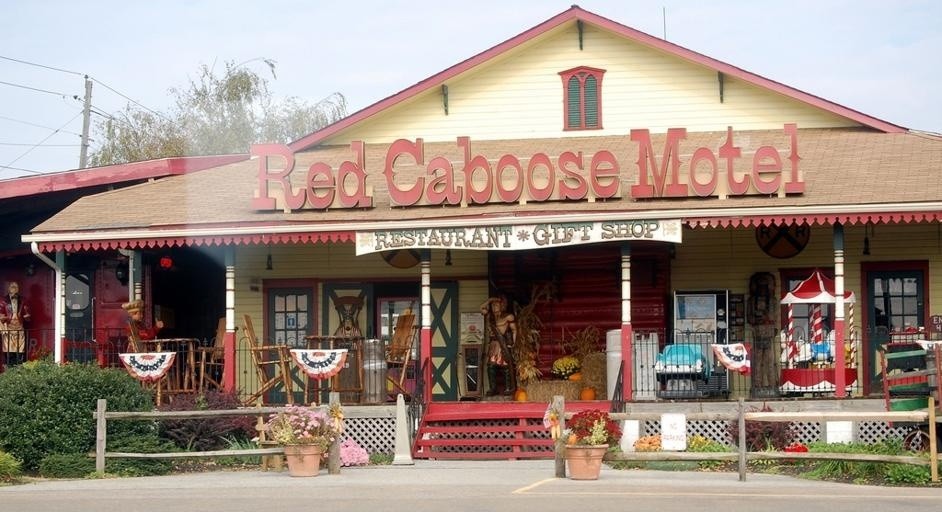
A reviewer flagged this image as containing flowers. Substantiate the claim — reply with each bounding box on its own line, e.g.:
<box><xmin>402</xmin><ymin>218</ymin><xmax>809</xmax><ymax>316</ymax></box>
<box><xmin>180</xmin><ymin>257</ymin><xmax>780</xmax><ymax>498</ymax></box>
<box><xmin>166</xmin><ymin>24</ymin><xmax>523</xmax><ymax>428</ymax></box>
<box><xmin>267</xmin><ymin>405</ymin><xmax>338</xmax><ymax>444</ymax></box>
<box><xmin>562</xmin><ymin>409</ymin><xmax>623</xmax><ymax>445</ymax></box>
<box><xmin>550</xmin><ymin>356</ymin><xmax>581</xmax><ymax>378</ymax></box>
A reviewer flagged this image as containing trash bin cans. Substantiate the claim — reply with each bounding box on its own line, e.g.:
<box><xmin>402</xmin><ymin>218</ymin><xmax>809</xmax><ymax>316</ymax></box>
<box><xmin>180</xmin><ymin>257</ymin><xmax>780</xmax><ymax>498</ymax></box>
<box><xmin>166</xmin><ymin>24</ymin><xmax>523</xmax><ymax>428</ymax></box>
<box><xmin>730</xmin><ymin>359</ymin><xmax>752</xmax><ymax>401</ymax></box>
<box><xmin>362</xmin><ymin>338</ymin><xmax>388</xmax><ymax>405</ymax></box>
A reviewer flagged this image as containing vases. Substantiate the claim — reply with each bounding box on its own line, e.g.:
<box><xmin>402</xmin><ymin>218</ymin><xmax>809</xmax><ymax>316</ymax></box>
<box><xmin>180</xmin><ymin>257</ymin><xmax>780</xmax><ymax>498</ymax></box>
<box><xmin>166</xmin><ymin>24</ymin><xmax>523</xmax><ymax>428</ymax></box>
<box><xmin>566</xmin><ymin>372</ymin><xmax>583</xmax><ymax>382</ymax></box>
<box><xmin>282</xmin><ymin>443</ymin><xmax>322</xmax><ymax>476</ymax></box>
<box><xmin>565</xmin><ymin>442</ymin><xmax>609</xmax><ymax>481</ymax></box>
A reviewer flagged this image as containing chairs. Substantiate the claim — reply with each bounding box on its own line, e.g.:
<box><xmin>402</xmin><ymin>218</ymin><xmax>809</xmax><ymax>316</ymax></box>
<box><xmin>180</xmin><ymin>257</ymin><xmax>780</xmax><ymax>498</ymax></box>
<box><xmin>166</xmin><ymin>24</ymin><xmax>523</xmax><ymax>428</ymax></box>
<box><xmin>384</xmin><ymin>311</ymin><xmax>416</xmax><ymax>401</ymax></box>
<box><xmin>241</xmin><ymin>313</ymin><xmax>297</xmax><ymax>406</ymax></box>
<box><xmin>124</xmin><ymin>318</ymin><xmax>176</xmax><ymax>405</ymax></box>
<box><xmin>255</xmin><ymin>402</ymin><xmax>288</xmax><ymax>472</ymax></box>
<box><xmin>186</xmin><ymin>316</ymin><xmax>230</xmax><ymax>402</ymax></box>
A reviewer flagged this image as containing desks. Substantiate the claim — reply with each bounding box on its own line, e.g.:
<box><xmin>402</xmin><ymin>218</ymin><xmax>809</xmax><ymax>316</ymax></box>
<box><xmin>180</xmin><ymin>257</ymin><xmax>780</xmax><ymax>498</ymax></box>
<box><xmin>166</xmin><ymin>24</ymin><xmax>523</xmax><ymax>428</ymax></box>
<box><xmin>149</xmin><ymin>337</ymin><xmax>201</xmax><ymax>410</ymax></box>
<box><xmin>298</xmin><ymin>334</ymin><xmax>367</xmax><ymax>405</ymax></box>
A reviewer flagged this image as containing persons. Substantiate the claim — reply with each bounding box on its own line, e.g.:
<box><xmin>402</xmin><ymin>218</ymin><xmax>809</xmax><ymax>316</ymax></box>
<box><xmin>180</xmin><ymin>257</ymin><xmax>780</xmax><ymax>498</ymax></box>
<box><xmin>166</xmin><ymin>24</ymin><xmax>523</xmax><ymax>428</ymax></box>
<box><xmin>479</xmin><ymin>296</ymin><xmax>517</xmax><ymax>396</ymax></box>
<box><xmin>121</xmin><ymin>300</ymin><xmax>164</xmax><ymax>354</ymax></box>
<box><xmin>0</xmin><ymin>281</ymin><xmax>31</xmax><ymax>367</ymax></box>
<box><xmin>334</xmin><ymin>311</ymin><xmax>361</xmax><ymax>349</ymax></box>
